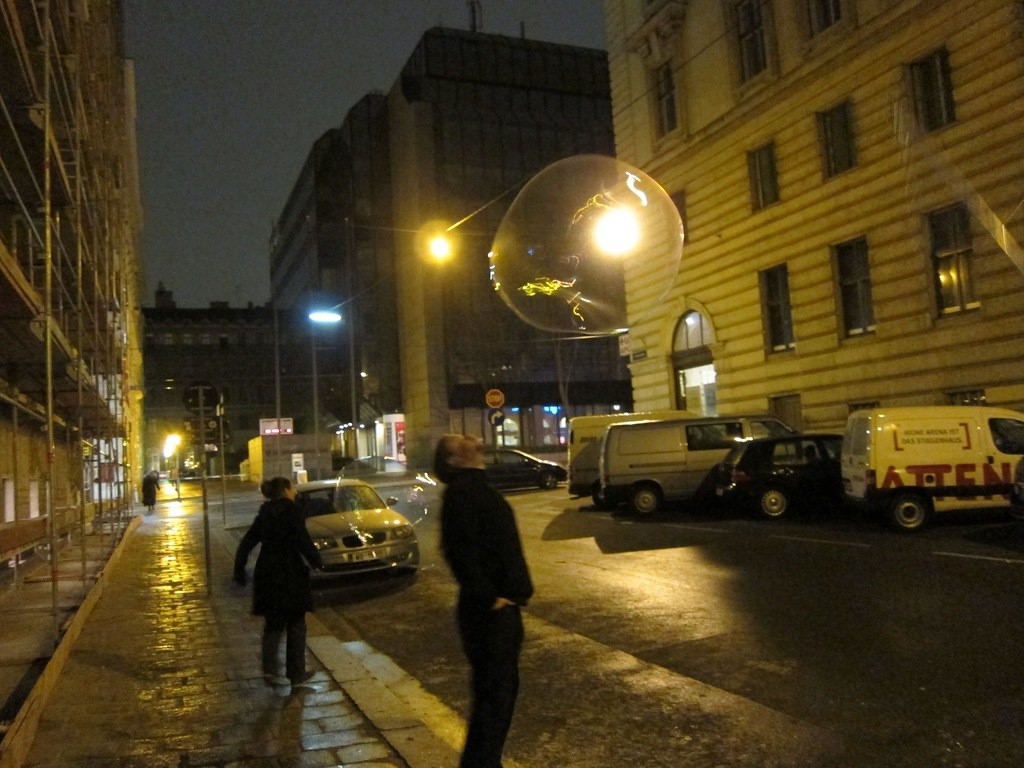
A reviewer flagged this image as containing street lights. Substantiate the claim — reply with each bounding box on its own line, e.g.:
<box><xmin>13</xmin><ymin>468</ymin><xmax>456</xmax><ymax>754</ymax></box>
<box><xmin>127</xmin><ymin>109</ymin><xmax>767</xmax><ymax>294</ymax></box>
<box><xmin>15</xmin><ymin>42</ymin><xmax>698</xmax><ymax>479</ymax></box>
<box><xmin>172</xmin><ymin>435</ymin><xmax>182</xmax><ymax>502</ymax></box>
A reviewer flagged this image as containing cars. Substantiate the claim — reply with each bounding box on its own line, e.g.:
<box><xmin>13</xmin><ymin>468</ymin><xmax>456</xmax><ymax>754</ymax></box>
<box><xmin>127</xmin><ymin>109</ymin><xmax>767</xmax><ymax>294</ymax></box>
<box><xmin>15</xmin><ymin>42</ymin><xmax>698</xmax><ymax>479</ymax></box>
<box><xmin>288</xmin><ymin>478</ymin><xmax>420</xmax><ymax>588</ymax></box>
<box><xmin>483</xmin><ymin>448</ymin><xmax>568</xmax><ymax>491</ymax></box>
<box><xmin>715</xmin><ymin>429</ymin><xmax>846</xmax><ymax>523</ymax></box>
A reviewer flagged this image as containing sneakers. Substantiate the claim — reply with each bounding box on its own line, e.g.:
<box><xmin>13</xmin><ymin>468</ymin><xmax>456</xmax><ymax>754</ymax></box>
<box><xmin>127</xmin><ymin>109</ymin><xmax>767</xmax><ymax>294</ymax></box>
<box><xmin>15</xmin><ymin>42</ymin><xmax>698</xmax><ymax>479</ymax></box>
<box><xmin>263</xmin><ymin>672</ymin><xmax>291</xmax><ymax>688</ymax></box>
<box><xmin>291</xmin><ymin>668</ymin><xmax>316</xmax><ymax>688</ymax></box>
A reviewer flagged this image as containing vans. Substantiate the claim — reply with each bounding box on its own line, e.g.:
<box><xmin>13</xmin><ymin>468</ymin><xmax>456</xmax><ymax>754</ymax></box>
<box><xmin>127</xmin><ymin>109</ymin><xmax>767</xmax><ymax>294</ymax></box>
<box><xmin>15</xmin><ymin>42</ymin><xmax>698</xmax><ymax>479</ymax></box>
<box><xmin>837</xmin><ymin>404</ymin><xmax>1024</xmax><ymax>533</ymax></box>
<box><xmin>599</xmin><ymin>411</ymin><xmax>835</xmax><ymax>522</ymax></box>
<box><xmin>567</xmin><ymin>409</ymin><xmax>738</xmax><ymax>513</ymax></box>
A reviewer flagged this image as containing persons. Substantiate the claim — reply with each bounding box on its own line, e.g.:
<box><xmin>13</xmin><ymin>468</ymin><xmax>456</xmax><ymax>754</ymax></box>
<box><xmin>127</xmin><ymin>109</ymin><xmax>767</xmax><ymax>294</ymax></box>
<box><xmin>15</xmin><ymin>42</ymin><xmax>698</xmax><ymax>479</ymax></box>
<box><xmin>232</xmin><ymin>478</ymin><xmax>322</xmax><ymax>689</ymax></box>
<box><xmin>434</xmin><ymin>433</ymin><xmax>534</xmax><ymax>768</ymax></box>
<box><xmin>142</xmin><ymin>470</ymin><xmax>160</xmax><ymax>515</ymax></box>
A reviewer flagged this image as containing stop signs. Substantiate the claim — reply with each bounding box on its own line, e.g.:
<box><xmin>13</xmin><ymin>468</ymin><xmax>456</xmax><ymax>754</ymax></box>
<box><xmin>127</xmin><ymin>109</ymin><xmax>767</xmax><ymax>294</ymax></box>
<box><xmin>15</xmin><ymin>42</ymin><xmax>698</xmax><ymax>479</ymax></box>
<box><xmin>486</xmin><ymin>389</ymin><xmax>505</xmax><ymax>410</ymax></box>
